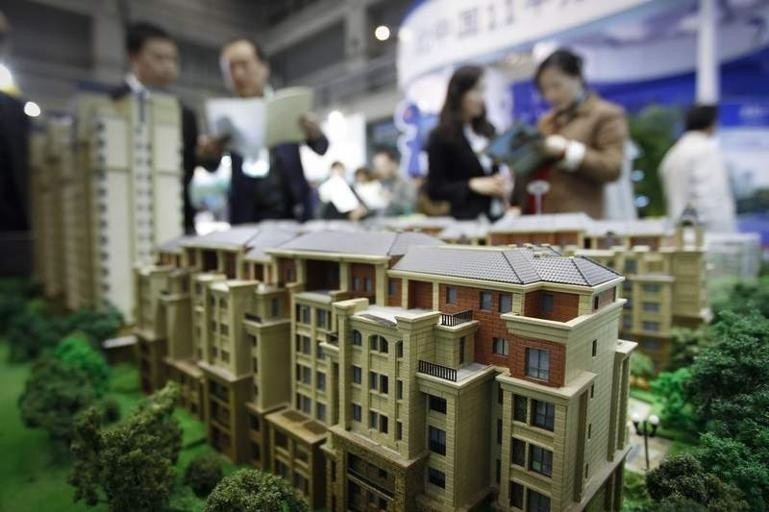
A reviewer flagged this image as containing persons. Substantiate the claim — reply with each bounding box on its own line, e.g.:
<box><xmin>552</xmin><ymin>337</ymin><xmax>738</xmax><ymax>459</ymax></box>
<box><xmin>107</xmin><ymin>22</ymin><xmax>200</xmax><ymax>238</ymax></box>
<box><xmin>192</xmin><ymin>37</ymin><xmax>329</xmax><ymax>223</ymax></box>
<box><xmin>525</xmin><ymin>49</ymin><xmax>630</xmax><ymax>219</ymax></box>
<box><xmin>316</xmin><ymin>142</ymin><xmax>426</xmax><ymax>228</ymax></box>
<box><xmin>420</xmin><ymin>63</ymin><xmax>516</xmax><ymax>220</ymax></box>
<box><xmin>658</xmin><ymin>101</ymin><xmax>742</xmax><ymax>230</ymax></box>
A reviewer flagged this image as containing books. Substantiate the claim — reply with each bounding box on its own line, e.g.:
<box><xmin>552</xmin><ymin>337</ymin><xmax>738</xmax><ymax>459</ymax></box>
<box><xmin>205</xmin><ymin>86</ymin><xmax>317</xmax><ymax>156</ymax></box>
